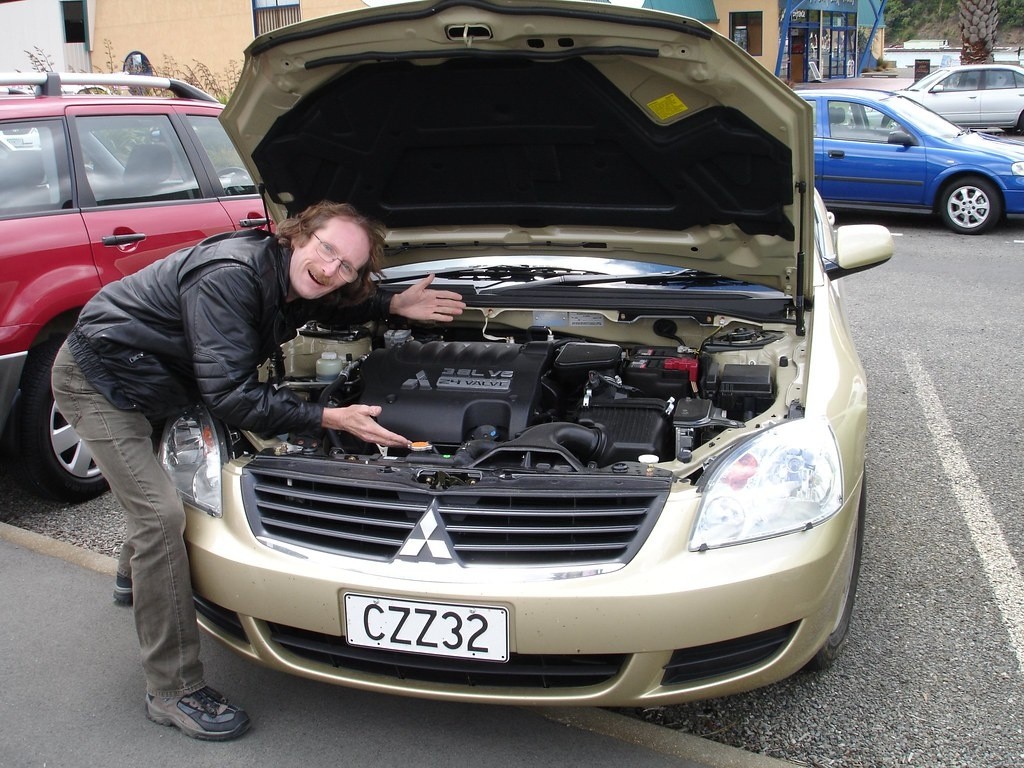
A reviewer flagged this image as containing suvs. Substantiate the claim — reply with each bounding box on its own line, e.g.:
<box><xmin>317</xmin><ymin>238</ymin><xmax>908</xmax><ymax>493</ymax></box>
<box><xmin>0</xmin><ymin>73</ymin><xmax>267</xmax><ymax>508</ymax></box>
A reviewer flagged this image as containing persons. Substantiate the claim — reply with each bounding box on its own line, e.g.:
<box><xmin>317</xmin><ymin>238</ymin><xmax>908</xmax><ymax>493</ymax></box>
<box><xmin>51</xmin><ymin>201</ymin><xmax>465</xmax><ymax>742</ymax></box>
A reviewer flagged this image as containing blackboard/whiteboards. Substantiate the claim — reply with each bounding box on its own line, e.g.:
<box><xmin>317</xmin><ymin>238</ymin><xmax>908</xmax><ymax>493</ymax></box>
<box><xmin>914</xmin><ymin>59</ymin><xmax>931</xmax><ymax>83</ymax></box>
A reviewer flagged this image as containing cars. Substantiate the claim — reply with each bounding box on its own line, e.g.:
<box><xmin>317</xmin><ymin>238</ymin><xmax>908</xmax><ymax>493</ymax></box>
<box><xmin>786</xmin><ymin>88</ymin><xmax>1023</xmax><ymax>239</ymax></box>
<box><xmin>155</xmin><ymin>0</ymin><xmax>898</xmax><ymax>717</ymax></box>
<box><xmin>893</xmin><ymin>63</ymin><xmax>1024</xmax><ymax>137</ymax></box>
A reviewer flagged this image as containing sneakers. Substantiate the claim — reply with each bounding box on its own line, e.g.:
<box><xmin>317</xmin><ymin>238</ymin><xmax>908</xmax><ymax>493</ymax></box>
<box><xmin>113</xmin><ymin>575</ymin><xmax>134</xmax><ymax>604</ymax></box>
<box><xmin>145</xmin><ymin>686</ymin><xmax>254</xmax><ymax>741</ymax></box>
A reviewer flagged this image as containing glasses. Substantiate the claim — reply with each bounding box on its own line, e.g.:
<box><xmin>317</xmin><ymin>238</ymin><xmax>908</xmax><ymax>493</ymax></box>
<box><xmin>311</xmin><ymin>231</ymin><xmax>358</xmax><ymax>283</ymax></box>
